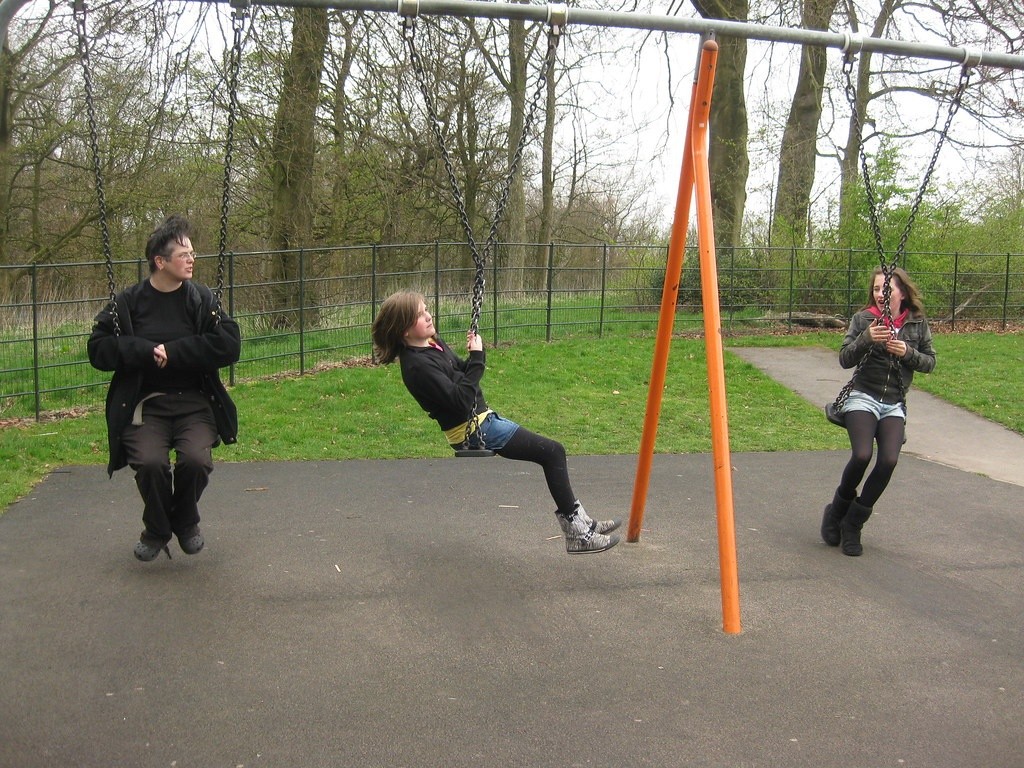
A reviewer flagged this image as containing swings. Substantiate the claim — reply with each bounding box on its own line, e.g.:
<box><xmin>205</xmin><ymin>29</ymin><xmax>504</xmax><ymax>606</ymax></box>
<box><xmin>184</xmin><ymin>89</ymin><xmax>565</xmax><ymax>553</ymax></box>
<box><xmin>826</xmin><ymin>44</ymin><xmax>974</xmax><ymax>447</ymax></box>
<box><xmin>70</xmin><ymin>1</ymin><xmax>248</xmax><ymax>448</ymax></box>
<box><xmin>400</xmin><ymin>16</ymin><xmax>560</xmax><ymax>458</ymax></box>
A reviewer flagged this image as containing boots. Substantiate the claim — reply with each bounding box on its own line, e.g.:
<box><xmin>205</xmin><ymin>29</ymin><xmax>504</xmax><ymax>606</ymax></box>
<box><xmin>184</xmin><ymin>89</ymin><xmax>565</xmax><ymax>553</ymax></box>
<box><xmin>820</xmin><ymin>486</ymin><xmax>858</xmax><ymax>546</ymax></box>
<box><xmin>558</xmin><ymin>503</ymin><xmax>621</xmax><ymax>554</ymax></box>
<box><xmin>839</xmin><ymin>498</ymin><xmax>873</xmax><ymax>556</ymax></box>
<box><xmin>554</xmin><ymin>498</ymin><xmax>623</xmax><ymax>534</ymax></box>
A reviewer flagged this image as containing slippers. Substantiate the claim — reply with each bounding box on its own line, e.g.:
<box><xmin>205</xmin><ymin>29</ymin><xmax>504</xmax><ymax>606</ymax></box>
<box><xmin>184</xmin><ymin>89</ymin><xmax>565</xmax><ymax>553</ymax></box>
<box><xmin>177</xmin><ymin>532</ymin><xmax>205</xmax><ymax>555</ymax></box>
<box><xmin>133</xmin><ymin>532</ymin><xmax>174</xmax><ymax>562</ymax></box>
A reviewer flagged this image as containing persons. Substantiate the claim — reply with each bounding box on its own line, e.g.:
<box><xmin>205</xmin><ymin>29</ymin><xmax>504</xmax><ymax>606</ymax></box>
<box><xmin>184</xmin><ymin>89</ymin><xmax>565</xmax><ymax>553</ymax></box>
<box><xmin>372</xmin><ymin>292</ymin><xmax>622</xmax><ymax>554</ymax></box>
<box><xmin>820</xmin><ymin>265</ymin><xmax>938</xmax><ymax>554</ymax></box>
<box><xmin>87</xmin><ymin>214</ymin><xmax>242</xmax><ymax>565</ymax></box>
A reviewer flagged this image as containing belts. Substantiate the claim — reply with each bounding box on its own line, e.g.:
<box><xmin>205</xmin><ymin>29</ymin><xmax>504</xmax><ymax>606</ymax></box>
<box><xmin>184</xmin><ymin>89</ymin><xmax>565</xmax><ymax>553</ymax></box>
<box><xmin>130</xmin><ymin>391</ymin><xmax>168</xmax><ymax>426</ymax></box>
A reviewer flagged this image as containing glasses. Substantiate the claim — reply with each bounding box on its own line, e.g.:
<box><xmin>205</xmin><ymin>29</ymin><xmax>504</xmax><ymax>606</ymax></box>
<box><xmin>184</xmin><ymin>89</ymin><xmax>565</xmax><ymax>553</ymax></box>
<box><xmin>167</xmin><ymin>251</ymin><xmax>197</xmax><ymax>260</ymax></box>
